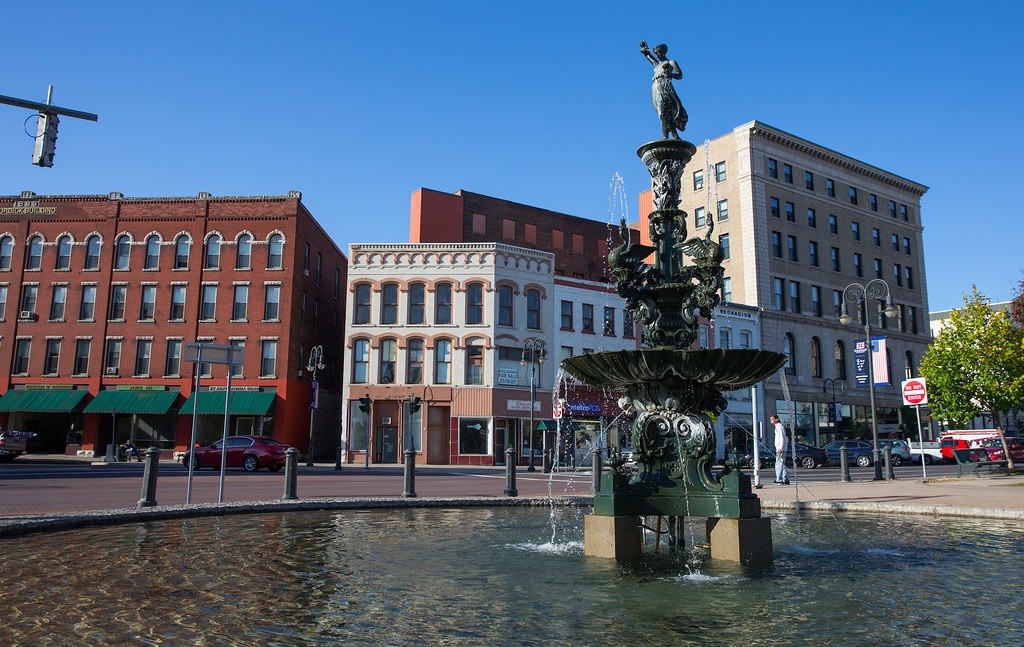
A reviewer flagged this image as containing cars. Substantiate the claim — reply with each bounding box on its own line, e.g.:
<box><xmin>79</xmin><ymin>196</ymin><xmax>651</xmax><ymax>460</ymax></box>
<box><xmin>182</xmin><ymin>436</ymin><xmax>291</xmax><ymax>473</ymax></box>
<box><xmin>866</xmin><ymin>438</ymin><xmax>911</xmax><ymax>466</ymax></box>
<box><xmin>970</xmin><ymin>437</ymin><xmax>1024</xmax><ymax>463</ymax></box>
<box><xmin>821</xmin><ymin>440</ymin><xmax>874</xmax><ymax>468</ymax></box>
<box><xmin>745</xmin><ymin>443</ymin><xmax>828</xmax><ymax>469</ymax></box>
<box><xmin>928</xmin><ymin>440</ymin><xmax>970</xmax><ymax>463</ymax></box>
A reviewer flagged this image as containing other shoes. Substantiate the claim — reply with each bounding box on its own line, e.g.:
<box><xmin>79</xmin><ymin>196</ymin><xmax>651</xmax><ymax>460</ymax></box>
<box><xmin>772</xmin><ymin>481</ymin><xmax>791</xmax><ymax>485</ymax></box>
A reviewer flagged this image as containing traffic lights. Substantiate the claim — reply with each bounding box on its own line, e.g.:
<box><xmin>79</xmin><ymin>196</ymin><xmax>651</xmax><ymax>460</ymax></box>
<box><xmin>358</xmin><ymin>398</ymin><xmax>369</xmax><ymax>413</ymax></box>
<box><xmin>411</xmin><ymin>397</ymin><xmax>421</xmax><ymax>412</ymax></box>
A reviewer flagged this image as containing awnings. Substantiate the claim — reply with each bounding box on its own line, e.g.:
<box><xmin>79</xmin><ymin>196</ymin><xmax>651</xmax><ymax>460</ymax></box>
<box><xmin>0</xmin><ymin>389</ymin><xmax>87</xmax><ymax>413</ymax></box>
<box><xmin>177</xmin><ymin>390</ymin><xmax>276</xmax><ymax>416</ymax></box>
<box><xmin>83</xmin><ymin>390</ymin><xmax>179</xmax><ymax>415</ymax></box>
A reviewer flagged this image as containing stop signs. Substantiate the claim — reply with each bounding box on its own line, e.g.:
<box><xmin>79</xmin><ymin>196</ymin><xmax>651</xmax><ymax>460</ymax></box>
<box><xmin>901</xmin><ymin>377</ymin><xmax>928</xmax><ymax>406</ymax></box>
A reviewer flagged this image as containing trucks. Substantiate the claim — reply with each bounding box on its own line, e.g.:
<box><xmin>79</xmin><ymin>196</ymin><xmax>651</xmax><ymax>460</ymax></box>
<box><xmin>893</xmin><ymin>438</ymin><xmax>944</xmax><ymax>465</ymax></box>
<box><xmin>0</xmin><ymin>431</ymin><xmax>27</xmax><ymax>461</ymax></box>
<box><xmin>936</xmin><ymin>429</ymin><xmax>1005</xmax><ymax>447</ymax></box>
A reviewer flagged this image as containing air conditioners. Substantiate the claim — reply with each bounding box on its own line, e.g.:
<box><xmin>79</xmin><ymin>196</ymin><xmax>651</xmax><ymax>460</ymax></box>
<box><xmin>107</xmin><ymin>367</ymin><xmax>118</xmax><ymax>373</ymax></box>
<box><xmin>21</xmin><ymin>311</ymin><xmax>32</xmax><ymax>319</ymax></box>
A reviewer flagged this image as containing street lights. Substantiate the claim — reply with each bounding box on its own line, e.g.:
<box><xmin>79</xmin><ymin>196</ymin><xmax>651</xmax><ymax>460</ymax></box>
<box><xmin>836</xmin><ymin>278</ymin><xmax>900</xmax><ymax>481</ymax></box>
<box><xmin>822</xmin><ymin>377</ymin><xmax>846</xmax><ymax>440</ymax></box>
<box><xmin>305</xmin><ymin>345</ymin><xmax>327</xmax><ymax>466</ymax></box>
<box><xmin>520</xmin><ymin>337</ymin><xmax>548</xmax><ymax>472</ymax></box>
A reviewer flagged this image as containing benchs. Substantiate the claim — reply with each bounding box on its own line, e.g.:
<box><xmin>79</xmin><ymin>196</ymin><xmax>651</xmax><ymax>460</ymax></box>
<box><xmin>952</xmin><ymin>448</ymin><xmax>1010</xmax><ymax>478</ymax></box>
<box><xmin>120</xmin><ymin>445</ymin><xmax>140</xmax><ymax>462</ymax></box>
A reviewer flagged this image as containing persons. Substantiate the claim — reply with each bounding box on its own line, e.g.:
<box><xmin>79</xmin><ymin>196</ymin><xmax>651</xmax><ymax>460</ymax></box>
<box><xmin>640</xmin><ymin>40</ymin><xmax>688</xmax><ymax>139</ymax></box>
<box><xmin>769</xmin><ymin>415</ymin><xmax>790</xmax><ymax>485</ymax></box>
<box><xmin>120</xmin><ymin>439</ymin><xmax>143</xmax><ymax>462</ymax></box>
